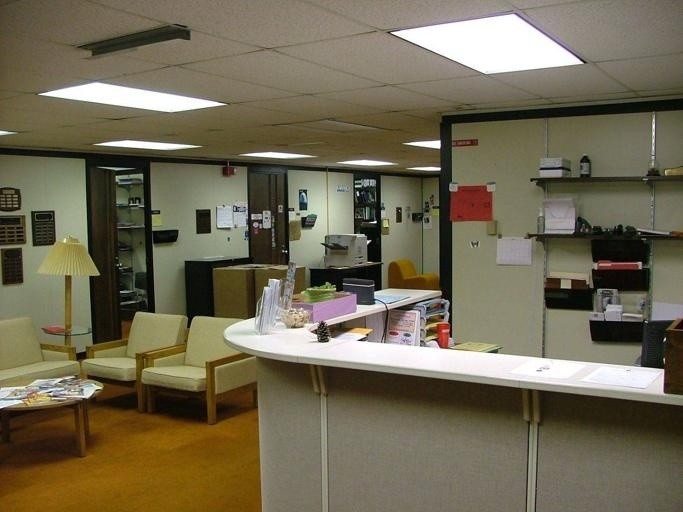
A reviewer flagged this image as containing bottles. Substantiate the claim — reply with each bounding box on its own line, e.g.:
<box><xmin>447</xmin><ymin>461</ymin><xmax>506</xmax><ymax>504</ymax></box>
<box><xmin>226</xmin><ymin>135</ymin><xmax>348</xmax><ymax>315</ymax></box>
<box><xmin>536</xmin><ymin>207</ymin><xmax>545</xmax><ymax>234</ymax></box>
<box><xmin>579</xmin><ymin>154</ymin><xmax>592</xmax><ymax>177</ymax></box>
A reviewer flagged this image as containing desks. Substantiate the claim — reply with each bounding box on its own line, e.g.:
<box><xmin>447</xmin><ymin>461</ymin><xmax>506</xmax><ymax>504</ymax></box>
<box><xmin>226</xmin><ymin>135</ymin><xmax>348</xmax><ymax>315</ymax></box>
<box><xmin>307</xmin><ymin>261</ymin><xmax>384</xmax><ymax>292</ymax></box>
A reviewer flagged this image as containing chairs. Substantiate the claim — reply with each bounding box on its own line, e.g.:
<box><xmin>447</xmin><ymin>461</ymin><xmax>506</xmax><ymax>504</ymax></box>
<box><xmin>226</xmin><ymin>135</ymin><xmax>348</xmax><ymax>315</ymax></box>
<box><xmin>79</xmin><ymin>312</ymin><xmax>189</xmax><ymax>413</ymax></box>
<box><xmin>0</xmin><ymin>316</ymin><xmax>79</xmax><ymax>390</ymax></box>
<box><xmin>142</xmin><ymin>316</ymin><xmax>258</xmax><ymax>426</ymax></box>
<box><xmin>388</xmin><ymin>260</ymin><xmax>441</xmax><ymax>292</ymax></box>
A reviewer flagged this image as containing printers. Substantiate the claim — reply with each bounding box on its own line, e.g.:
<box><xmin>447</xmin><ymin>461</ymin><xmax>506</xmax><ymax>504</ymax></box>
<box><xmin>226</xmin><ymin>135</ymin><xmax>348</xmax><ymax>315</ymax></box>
<box><xmin>321</xmin><ymin>232</ymin><xmax>369</xmax><ymax>266</ymax></box>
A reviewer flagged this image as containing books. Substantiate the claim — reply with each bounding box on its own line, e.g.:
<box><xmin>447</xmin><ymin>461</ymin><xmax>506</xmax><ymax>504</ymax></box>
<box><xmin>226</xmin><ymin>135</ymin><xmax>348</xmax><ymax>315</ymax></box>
<box><xmin>0</xmin><ymin>374</ymin><xmax>104</xmax><ymax>408</ymax></box>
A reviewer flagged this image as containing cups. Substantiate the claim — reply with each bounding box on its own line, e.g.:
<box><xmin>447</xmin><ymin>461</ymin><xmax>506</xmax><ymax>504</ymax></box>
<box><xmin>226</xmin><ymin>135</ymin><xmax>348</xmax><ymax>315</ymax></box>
<box><xmin>436</xmin><ymin>322</ymin><xmax>450</xmax><ymax>347</ymax></box>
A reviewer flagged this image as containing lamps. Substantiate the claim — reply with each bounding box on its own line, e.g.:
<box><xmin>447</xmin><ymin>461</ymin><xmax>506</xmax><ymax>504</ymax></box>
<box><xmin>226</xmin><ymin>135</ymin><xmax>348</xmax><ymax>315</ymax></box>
<box><xmin>35</xmin><ymin>235</ymin><xmax>101</xmax><ymax>344</ymax></box>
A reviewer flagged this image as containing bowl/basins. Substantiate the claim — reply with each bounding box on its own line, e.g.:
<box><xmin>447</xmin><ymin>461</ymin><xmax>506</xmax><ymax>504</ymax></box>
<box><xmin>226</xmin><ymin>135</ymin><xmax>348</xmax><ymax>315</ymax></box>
<box><xmin>282</xmin><ymin>309</ymin><xmax>312</xmax><ymax>328</ymax></box>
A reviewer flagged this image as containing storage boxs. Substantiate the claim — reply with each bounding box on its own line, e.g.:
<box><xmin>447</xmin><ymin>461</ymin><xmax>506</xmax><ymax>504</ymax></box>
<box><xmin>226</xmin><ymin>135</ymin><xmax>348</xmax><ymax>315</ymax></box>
<box><xmin>256</xmin><ymin>265</ymin><xmax>305</xmax><ymax>302</ymax></box>
<box><xmin>212</xmin><ymin>265</ymin><xmax>272</xmax><ymax>319</ymax></box>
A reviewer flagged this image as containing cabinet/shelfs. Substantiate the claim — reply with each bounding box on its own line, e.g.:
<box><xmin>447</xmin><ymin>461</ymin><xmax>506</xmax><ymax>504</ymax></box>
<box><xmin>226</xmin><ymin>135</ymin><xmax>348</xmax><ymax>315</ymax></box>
<box><xmin>528</xmin><ymin>176</ymin><xmax>682</xmax><ymax>240</ymax></box>
<box><xmin>185</xmin><ymin>257</ymin><xmax>253</xmax><ymax>328</ymax></box>
